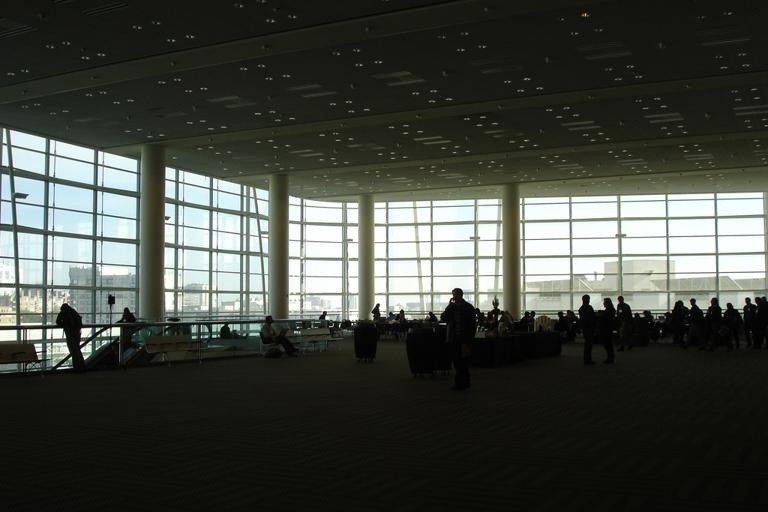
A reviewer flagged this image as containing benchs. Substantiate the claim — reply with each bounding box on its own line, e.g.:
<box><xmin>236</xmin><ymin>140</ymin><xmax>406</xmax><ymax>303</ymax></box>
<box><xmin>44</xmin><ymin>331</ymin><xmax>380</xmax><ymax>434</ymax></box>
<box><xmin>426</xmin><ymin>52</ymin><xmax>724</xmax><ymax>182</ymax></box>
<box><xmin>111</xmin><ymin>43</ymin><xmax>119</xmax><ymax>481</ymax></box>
<box><xmin>258</xmin><ymin>327</ymin><xmax>345</xmax><ymax>354</ymax></box>
<box><xmin>0</xmin><ymin>342</ymin><xmax>48</xmax><ymax>375</ymax></box>
<box><xmin>143</xmin><ymin>334</ymin><xmax>210</xmax><ymax>365</ymax></box>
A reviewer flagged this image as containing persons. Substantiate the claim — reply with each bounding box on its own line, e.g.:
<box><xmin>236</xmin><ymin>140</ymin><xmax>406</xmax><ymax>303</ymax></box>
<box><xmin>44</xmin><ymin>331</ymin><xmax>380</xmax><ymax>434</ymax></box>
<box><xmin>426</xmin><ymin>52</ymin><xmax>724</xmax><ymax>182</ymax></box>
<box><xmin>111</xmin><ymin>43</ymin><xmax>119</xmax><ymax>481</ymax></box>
<box><xmin>318</xmin><ymin>311</ymin><xmax>327</xmax><ymax>328</ymax></box>
<box><xmin>56</xmin><ymin>303</ymin><xmax>87</xmax><ymax>373</ymax></box>
<box><xmin>371</xmin><ymin>288</ymin><xmax>767</xmax><ymax>390</ymax></box>
<box><xmin>220</xmin><ymin>322</ymin><xmax>249</xmax><ymax>340</ymax></box>
<box><xmin>260</xmin><ymin>316</ymin><xmax>299</xmax><ymax>357</ymax></box>
<box><xmin>116</xmin><ymin>308</ymin><xmax>137</xmax><ymax>345</ymax></box>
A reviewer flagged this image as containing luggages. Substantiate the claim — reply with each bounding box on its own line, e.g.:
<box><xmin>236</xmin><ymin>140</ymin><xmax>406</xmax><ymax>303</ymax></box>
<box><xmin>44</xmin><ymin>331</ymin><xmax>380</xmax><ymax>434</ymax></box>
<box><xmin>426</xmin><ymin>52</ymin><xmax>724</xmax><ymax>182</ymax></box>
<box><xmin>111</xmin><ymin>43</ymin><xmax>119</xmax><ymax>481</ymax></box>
<box><xmin>405</xmin><ymin>323</ymin><xmax>452</xmax><ymax>380</ymax></box>
<box><xmin>353</xmin><ymin>321</ymin><xmax>377</xmax><ymax>363</ymax></box>
